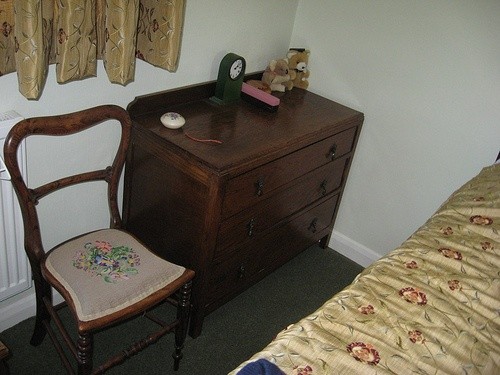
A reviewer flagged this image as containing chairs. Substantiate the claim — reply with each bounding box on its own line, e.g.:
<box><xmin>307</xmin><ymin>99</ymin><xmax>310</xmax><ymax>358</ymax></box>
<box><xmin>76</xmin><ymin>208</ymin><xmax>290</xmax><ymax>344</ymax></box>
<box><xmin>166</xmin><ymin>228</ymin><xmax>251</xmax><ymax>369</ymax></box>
<box><xmin>4</xmin><ymin>105</ymin><xmax>195</xmax><ymax>375</ymax></box>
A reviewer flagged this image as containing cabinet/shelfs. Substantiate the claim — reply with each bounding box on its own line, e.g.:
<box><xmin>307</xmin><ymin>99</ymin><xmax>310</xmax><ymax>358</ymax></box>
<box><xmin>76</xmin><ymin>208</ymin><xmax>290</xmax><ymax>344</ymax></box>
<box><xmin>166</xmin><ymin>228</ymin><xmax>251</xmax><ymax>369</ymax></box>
<box><xmin>123</xmin><ymin>71</ymin><xmax>364</xmax><ymax>339</ymax></box>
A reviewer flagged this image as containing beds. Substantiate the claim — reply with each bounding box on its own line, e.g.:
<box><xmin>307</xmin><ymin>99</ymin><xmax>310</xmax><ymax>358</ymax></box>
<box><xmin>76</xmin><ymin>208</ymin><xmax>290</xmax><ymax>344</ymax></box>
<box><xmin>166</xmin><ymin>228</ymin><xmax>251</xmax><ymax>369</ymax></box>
<box><xmin>227</xmin><ymin>152</ymin><xmax>499</xmax><ymax>375</ymax></box>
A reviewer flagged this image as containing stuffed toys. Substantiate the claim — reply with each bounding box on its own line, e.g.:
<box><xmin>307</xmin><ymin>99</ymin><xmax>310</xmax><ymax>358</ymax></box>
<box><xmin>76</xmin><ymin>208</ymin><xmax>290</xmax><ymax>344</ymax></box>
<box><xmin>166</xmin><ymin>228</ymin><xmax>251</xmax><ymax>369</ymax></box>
<box><xmin>284</xmin><ymin>48</ymin><xmax>310</xmax><ymax>90</ymax></box>
<box><xmin>262</xmin><ymin>58</ymin><xmax>290</xmax><ymax>92</ymax></box>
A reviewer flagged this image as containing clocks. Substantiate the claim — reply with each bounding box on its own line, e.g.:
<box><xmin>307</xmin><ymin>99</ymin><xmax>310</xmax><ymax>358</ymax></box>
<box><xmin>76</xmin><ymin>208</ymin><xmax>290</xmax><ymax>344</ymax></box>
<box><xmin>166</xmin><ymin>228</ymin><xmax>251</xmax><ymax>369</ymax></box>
<box><xmin>209</xmin><ymin>53</ymin><xmax>246</xmax><ymax>106</ymax></box>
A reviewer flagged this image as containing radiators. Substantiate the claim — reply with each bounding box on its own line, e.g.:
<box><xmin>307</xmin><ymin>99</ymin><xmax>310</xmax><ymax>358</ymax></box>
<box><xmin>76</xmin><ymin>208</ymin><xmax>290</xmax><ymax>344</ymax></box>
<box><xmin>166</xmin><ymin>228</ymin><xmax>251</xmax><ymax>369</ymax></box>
<box><xmin>0</xmin><ymin>111</ymin><xmax>32</xmax><ymax>302</ymax></box>
